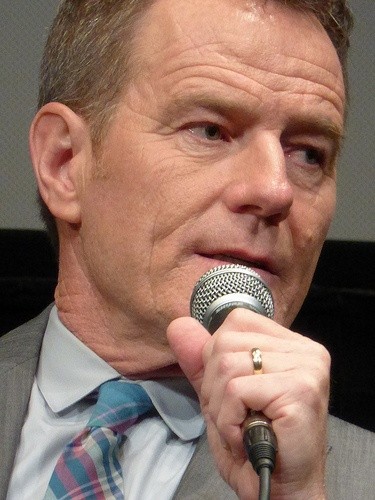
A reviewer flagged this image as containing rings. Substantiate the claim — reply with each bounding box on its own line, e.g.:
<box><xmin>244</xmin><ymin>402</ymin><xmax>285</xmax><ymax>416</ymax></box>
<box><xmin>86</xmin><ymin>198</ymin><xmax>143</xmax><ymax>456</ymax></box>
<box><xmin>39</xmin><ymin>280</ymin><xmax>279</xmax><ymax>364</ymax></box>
<box><xmin>251</xmin><ymin>348</ymin><xmax>263</xmax><ymax>375</ymax></box>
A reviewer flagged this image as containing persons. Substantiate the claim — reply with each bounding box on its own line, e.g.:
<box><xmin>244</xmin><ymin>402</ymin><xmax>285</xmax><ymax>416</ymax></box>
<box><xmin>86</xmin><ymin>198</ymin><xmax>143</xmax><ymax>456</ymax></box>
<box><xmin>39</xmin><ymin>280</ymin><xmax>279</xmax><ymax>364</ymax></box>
<box><xmin>0</xmin><ymin>0</ymin><xmax>374</xmax><ymax>500</ymax></box>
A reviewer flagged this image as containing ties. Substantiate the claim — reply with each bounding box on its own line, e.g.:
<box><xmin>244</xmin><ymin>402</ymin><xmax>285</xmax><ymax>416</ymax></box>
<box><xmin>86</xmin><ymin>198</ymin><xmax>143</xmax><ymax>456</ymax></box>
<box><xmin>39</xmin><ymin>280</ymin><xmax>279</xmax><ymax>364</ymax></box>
<box><xmin>43</xmin><ymin>380</ymin><xmax>154</xmax><ymax>500</ymax></box>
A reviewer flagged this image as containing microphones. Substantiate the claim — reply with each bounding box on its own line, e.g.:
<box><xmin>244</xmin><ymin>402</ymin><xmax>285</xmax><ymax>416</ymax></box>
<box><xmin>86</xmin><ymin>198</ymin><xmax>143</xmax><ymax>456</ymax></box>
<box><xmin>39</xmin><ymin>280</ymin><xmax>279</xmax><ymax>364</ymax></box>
<box><xmin>190</xmin><ymin>265</ymin><xmax>276</xmax><ymax>473</ymax></box>
